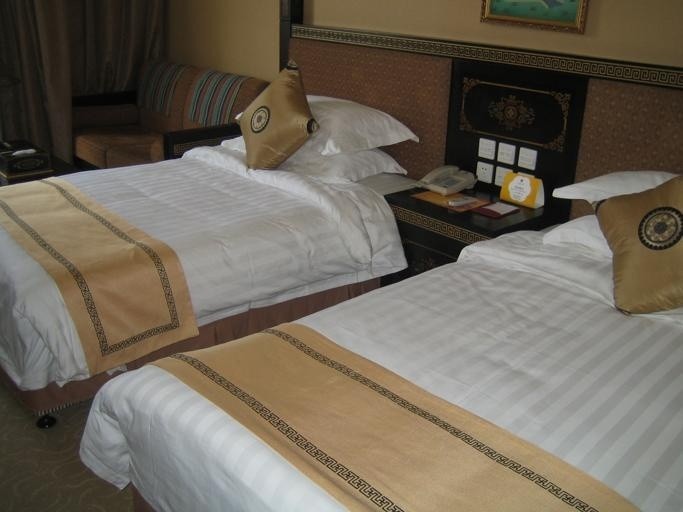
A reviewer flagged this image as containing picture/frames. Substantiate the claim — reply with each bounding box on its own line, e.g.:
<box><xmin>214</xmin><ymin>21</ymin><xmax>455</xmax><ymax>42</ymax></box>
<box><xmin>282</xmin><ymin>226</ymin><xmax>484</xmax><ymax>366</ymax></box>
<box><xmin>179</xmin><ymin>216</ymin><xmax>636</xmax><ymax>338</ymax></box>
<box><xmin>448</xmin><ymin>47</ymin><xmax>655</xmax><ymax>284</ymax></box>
<box><xmin>479</xmin><ymin>0</ymin><xmax>588</xmax><ymax>35</ymax></box>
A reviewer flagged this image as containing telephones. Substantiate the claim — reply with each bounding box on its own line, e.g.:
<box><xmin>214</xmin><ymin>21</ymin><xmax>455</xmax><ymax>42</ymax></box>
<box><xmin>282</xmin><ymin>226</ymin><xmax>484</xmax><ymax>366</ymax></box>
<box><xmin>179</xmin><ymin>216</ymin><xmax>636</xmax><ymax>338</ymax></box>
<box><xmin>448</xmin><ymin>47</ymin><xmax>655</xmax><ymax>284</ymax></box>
<box><xmin>416</xmin><ymin>165</ymin><xmax>475</xmax><ymax>197</ymax></box>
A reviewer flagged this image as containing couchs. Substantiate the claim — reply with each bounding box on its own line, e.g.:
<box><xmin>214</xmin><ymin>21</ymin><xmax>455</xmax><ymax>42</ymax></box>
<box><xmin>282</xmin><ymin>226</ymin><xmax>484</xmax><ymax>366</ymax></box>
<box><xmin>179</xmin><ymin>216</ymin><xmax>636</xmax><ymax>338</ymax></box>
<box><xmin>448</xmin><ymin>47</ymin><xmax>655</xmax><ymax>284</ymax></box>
<box><xmin>71</xmin><ymin>58</ymin><xmax>271</xmax><ymax>170</ymax></box>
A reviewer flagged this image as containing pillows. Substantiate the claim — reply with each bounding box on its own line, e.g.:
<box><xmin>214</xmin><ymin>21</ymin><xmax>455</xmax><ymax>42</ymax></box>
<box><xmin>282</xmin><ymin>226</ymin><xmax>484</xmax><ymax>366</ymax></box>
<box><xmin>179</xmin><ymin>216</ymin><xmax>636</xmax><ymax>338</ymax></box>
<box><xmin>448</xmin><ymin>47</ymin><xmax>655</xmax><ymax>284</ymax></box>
<box><xmin>542</xmin><ymin>169</ymin><xmax>683</xmax><ymax>316</ymax></box>
<box><xmin>221</xmin><ymin>59</ymin><xmax>421</xmax><ymax>185</ymax></box>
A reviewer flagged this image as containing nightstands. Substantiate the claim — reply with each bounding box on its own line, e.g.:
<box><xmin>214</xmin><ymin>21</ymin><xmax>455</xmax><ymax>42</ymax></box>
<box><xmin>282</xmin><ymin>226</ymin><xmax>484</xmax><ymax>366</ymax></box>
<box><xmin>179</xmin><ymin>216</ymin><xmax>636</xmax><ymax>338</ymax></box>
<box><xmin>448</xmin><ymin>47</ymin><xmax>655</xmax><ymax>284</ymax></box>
<box><xmin>380</xmin><ymin>180</ymin><xmax>570</xmax><ymax>288</ymax></box>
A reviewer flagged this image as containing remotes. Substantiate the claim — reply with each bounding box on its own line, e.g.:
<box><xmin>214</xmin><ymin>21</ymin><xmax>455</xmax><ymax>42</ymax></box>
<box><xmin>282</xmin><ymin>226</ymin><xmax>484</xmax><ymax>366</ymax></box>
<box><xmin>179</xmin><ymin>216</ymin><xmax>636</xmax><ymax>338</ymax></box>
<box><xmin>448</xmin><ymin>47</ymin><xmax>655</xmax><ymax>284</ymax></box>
<box><xmin>448</xmin><ymin>196</ymin><xmax>478</xmax><ymax>207</ymax></box>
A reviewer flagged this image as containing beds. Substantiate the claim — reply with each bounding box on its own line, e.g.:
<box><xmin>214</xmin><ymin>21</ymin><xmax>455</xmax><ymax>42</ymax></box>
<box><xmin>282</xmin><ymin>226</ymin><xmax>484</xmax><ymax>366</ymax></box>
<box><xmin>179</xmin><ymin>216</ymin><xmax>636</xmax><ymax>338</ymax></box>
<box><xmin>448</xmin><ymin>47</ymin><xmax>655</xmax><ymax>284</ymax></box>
<box><xmin>97</xmin><ymin>212</ymin><xmax>683</xmax><ymax>512</ymax></box>
<box><xmin>0</xmin><ymin>146</ymin><xmax>421</xmax><ymax>430</ymax></box>
